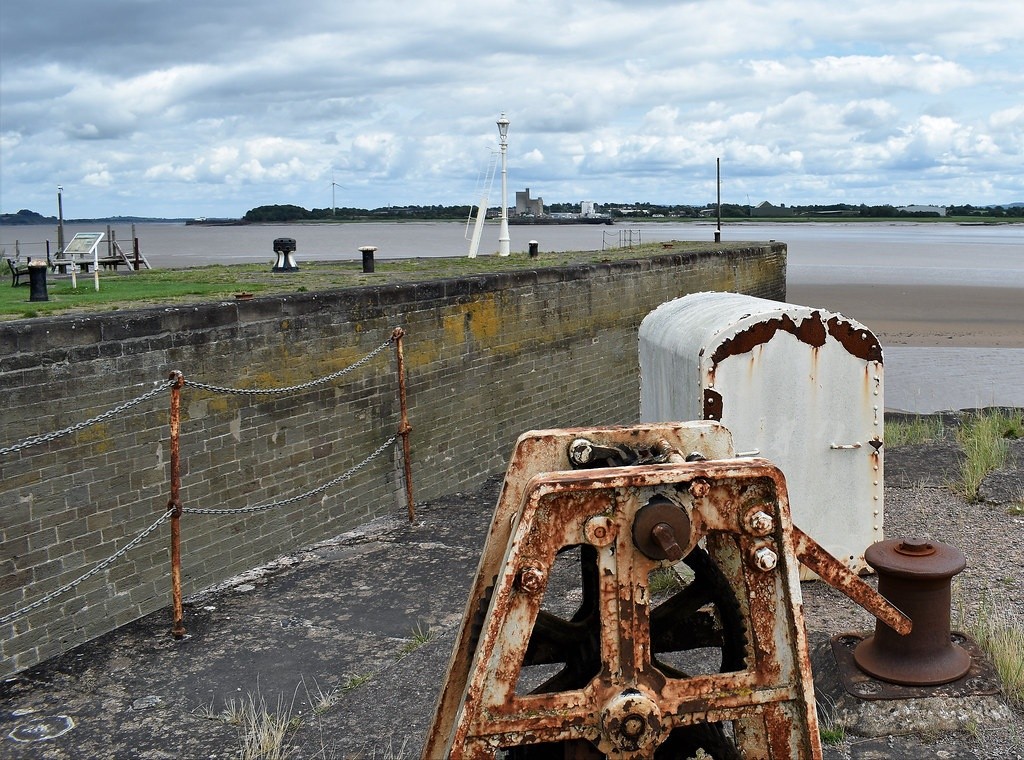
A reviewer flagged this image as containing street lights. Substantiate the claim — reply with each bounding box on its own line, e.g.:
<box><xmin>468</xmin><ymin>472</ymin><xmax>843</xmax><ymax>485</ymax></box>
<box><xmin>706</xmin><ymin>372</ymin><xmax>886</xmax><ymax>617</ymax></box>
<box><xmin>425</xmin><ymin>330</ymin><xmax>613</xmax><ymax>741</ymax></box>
<box><xmin>496</xmin><ymin>108</ymin><xmax>512</xmax><ymax>257</ymax></box>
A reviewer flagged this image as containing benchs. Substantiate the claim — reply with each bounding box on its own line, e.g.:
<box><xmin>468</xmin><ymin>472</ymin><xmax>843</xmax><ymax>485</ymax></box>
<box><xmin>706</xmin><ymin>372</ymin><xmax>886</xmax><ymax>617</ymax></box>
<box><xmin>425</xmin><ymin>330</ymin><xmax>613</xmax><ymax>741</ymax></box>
<box><xmin>7</xmin><ymin>258</ymin><xmax>30</xmax><ymax>286</ymax></box>
